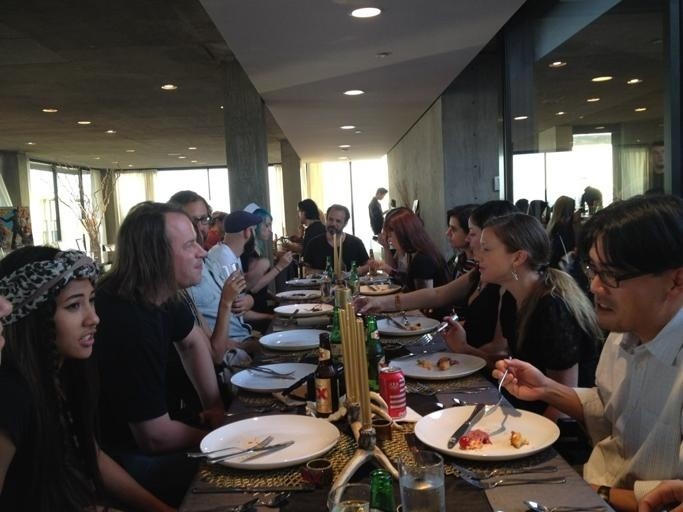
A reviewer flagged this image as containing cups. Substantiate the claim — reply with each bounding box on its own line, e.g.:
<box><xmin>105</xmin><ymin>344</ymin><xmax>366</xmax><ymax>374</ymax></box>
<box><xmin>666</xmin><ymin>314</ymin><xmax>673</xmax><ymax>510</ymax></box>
<box><xmin>397</xmin><ymin>448</ymin><xmax>448</xmax><ymax>512</ymax></box>
<box><xmin>300</xmin><ymin>456</ymin><xmax>332</xmax><ymax>487</ymax></box>
<box><xmin>328</xmin><ymin>481</ymin><xmax>370</xmax><ymax>511</ymax></box>
<box><xmin>221</xmin><ymin>263</ymin><xmax>240</xmax><ymax>299</ymax></box>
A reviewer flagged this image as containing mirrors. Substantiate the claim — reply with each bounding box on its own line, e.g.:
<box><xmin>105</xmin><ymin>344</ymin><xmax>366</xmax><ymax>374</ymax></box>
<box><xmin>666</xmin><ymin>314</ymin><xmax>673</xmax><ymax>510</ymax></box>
<box><xmin>509</xmin><ymin>3</ymin><xmax>674</xmax><ymax>261</ymax></box>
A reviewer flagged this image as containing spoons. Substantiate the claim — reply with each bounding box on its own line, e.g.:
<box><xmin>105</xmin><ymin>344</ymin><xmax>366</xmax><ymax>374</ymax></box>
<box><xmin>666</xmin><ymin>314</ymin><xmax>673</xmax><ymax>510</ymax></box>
<box><xmin>205</xmin><ymin>491</ymin><xmax>291</xmax><ymax>512</ymax></box>
<box><xmin>521</xmin><ymin>497</ymin><xmax>607</xmax><ymax>511</ymax></box>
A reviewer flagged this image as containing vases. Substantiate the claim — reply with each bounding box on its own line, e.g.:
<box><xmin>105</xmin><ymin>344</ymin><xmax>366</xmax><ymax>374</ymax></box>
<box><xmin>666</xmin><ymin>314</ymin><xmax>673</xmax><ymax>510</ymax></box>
<box><xmin>86</xmin><ymin>229</ymin><xmax>101</xmax><ymax>265</ymax></box>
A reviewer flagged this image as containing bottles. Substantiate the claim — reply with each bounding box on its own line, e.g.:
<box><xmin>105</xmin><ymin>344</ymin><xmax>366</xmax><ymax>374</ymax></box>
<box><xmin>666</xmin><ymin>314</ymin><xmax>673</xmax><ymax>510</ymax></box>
<box><xmin>295</xmin><ymin>252</ymin><xmax>309</xmax><ymax>279</ymax></box>
<box><xmin>366</xmin><ymin>315</ymin><xmax>385</xmax><ymax>393</ymax></box>
<box><xmin>369</xmin><ymin>469</ymin><xmax>397</xmax><ymax>512</ymax></box>
<box><xmin>323</xmin><ymin>256</ymin><xmax>334</xmax><ymax>284</ymax></box>
<box><xmin>348</xmin><ymin>260</ymin><xmax>360</xmax><ymax>298</ymax></box>
<box><xmin>331</xmin><ymin>287</ymin><xmax>353</xmax><ymax>342</ymax></box>
<box><xmin>368</xmin><ymin>248</ymin><xmax>377</xmax><ymax>278</ymax></box>
<box><xmin>314</xmin><ymin>332</ymin><xmax>339</xmax><ymax>417</ymax></box>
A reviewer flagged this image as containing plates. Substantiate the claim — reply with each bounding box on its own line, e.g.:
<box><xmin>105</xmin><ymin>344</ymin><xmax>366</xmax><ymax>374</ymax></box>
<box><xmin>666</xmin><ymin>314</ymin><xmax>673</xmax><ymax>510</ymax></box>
<box><xmin>229</xmin><ymin>363</ymin><xmax>317</xmax><ymax>393</ymax></box>
<box><xmin>258</xmin><ymin>329</ymin><xmax>331</xmax><ymax>351</ymax></box>
<box><xmin>389</xmin><ymin>352</ymin><xmax>487</xmax><ymax>380</ymax></box>
<box><xmin>198</xmin><ymin>414</ymin><xmax>341</xmax><ymax>470</ymax></box>
<box><xmin>272</xmin><ymin>278</ymin><xmax>334</xmax><ymax>317</ymax></box>
<box><xmin>377</xmin><ymin>316</ymin><xmax>441</xmax><ymax>336</ymax></box>
<box><xmin>411</xmin><ymin>404</ymin><xmax>559</xmax><ymax>459</ymax></box>
<box><xmin>358</xmin><ymin>275</ymin><xmax>401</xmax><ymax>296</ymax></box>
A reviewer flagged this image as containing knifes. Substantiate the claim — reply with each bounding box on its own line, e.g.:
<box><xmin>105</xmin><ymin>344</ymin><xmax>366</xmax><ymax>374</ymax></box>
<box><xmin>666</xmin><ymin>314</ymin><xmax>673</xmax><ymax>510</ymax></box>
<box><xmin>282</xmin><ymin>309</ymin><xmax>298</xmax><ymax>327</ymax></box>
<box><xmin>447</xmin><ymin>401</ymin><xmax>484</xmax><ymax>448</ymax></box>
<box><xmin>221</xmin><ymin>407</ymin><xmax>273</xmax><ymax>416</ymax></box>
<box><xmin>189</xmin><ymin>487</ymin><xmax>315</xmax><ymax>495</ymax></box>
<box><xmin>205</xmin><ymin>441</ymin><xmax>296</xmax><ymax>465</ymax></box>
<box><xmin>384</xmin><ymin>313</ymin><xmax>406</xmax><ymax>329</ymax></box>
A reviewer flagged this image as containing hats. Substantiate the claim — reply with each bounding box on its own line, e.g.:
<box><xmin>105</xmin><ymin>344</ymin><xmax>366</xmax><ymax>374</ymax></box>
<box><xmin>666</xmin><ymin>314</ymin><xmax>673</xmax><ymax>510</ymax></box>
<box><xmin>224</xmin><ymin>211</ymin><xmax>262</xmax><ymax>232</ymax></box>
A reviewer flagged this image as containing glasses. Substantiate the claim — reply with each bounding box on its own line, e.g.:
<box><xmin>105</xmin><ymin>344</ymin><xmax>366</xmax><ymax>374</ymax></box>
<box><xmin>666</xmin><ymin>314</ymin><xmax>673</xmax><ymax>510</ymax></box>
<box><xmin>193</xmin><ymin>216</ymin><xmax>211</xmax><ymax>225</ymax></box>
<box><xmin>582</xmin><ymin>263</ymin><xmax>647</xmax><ymax>287</ymax></box>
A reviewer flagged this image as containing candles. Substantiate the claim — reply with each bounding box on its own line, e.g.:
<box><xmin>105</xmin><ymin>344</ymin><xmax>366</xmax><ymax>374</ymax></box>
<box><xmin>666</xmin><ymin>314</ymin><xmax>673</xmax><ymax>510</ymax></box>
<box><xmin>354</xmin><ymin>317</ymin><xmax>373</xmax><ymax>429</ymax></box>
<box><xmin>344</xmin><ymin>297</ymin><xmax>361</xmax><ymax>405</ymax></box>
<box><xmin>331</xmin><ymin>230</ymin><xmax>344</xmax><ymax>277</ymax></box>
<box><xmin>336</xmin><ymin>306</ymin><xmax>352</xmax><ymax>398</ymax></box>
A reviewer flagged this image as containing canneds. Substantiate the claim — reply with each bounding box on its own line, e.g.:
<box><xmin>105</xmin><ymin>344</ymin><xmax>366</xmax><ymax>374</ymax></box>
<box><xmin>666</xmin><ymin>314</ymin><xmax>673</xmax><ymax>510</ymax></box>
<box><xmin>379</xmin><ymin>367</ymin><xmax>407</xmax><ymax>419</ymax></box>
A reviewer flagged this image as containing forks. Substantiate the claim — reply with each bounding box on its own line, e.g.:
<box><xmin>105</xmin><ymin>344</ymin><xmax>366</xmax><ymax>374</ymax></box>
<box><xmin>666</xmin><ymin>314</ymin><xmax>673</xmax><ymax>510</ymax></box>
<box><xmin>233</xmin><ymin>364</ymin><xmax>294</xmax><ymax>377</ymax></box>
<box><xmin>449</xmin><ymin>461</ymin><xmax>565</xmax><ymax>489</ymax></box>
<box><xmin>413</xmin><ymin>315</ymin><xmax>458</xmax><ymax>347</ymax></box>
<box><xmin>400</xmin><ymin>310</ymin><xmax>411</xmax><ymax>327</ymax></box>
<box><xmin>485</xmin><ymin>356</ymin><xmax>513</xmax><ymax>415</ymax></box>
<box><xmin>185</xmin><ymin>434</ymin><xmax>273</xmax><ymax>459</ymax></box>
